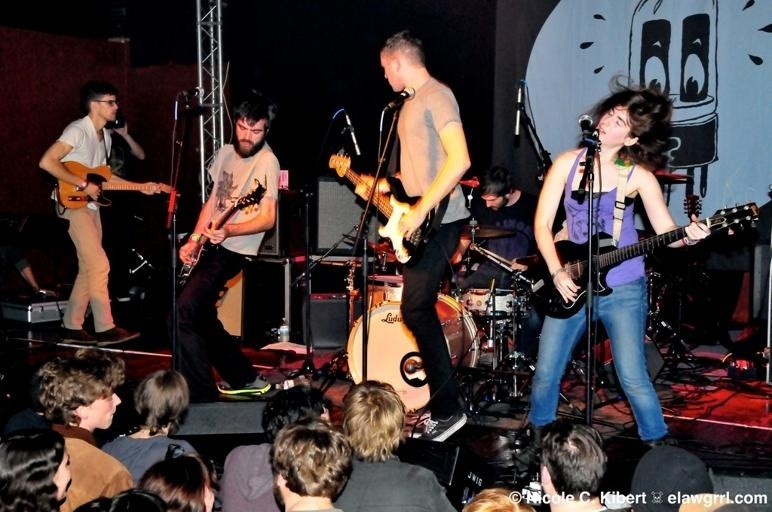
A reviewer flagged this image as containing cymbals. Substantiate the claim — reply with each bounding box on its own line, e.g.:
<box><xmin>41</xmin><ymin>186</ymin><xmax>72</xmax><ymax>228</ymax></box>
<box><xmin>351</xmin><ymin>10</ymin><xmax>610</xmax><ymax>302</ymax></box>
<box><xmin>467</xmin><ymin>226</ymin><xmax>518</xmax><ymax>240</ymax></box>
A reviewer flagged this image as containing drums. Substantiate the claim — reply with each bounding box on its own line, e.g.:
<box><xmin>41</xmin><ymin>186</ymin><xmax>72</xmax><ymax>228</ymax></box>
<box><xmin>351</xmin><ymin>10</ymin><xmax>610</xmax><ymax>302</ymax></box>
<box><xmin>345</xmin><ymin>286</ymin><xmax>482</xmax><ymax>423</ymax></box>
<box><xmin>462</xmin><ymin>288</ymin><xmax>520</xmax><ymax>322</ymax></box>
<box><xmin>366</xmin><ymin>273</ymin><xmax>450</xmax><ymax>310</ymax></box>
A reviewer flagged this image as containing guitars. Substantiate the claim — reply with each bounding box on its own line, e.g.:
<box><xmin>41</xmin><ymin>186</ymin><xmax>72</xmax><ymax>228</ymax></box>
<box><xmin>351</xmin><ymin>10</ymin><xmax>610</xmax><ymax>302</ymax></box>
<box><xmin>174</xmin><ymin>184</ymin><xmax>267</xmax><ymax>291</ymax></box>
<box><xmin>325</xmin><ymin>148</ymin><xmax>433</xmax><ymax>266</ymax></box>
<box><xmin>51</xmin><ymin>159</ymin><xmax>182</xmax><ymax>211</ymax></box>
<box><xmin>521</xmin><ymin>199</ymin><xmax>758</xmax><ymax>320</ymax></box>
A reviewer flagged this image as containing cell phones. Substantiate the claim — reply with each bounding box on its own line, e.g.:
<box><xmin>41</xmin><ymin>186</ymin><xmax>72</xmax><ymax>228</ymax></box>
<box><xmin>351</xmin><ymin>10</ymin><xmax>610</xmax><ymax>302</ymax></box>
<box><xmin>104</xmin><ymin>116</ymin><xmax>124</xmax><ymax>130</ymax></box>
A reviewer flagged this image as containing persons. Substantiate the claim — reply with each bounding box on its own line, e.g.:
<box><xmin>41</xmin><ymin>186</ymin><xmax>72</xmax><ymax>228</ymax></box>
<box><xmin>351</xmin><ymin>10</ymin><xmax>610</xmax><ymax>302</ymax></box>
<box><xmin>38</xmin><ymin>85</ymin><xmax>161</xmax><ymax>348</ymax></box>
<box><xmin>457</xmin><ymin>166</ymin><xmax>541</xmax><ymax>357</ymax></box>
<box><xmin>87</xmin><ymin>370</ymin><xmax>211</xmax><ymax>488</ymax></box>
<box><xmin>270</xmin><ymin>424</ymin><xmax>351</xmax><ymax>512</ymax></box>
<box><xmin>133</xmin><ymin>442</ymin><xmax>213</xmax><ymax>512</ymax></box>
<box><xmin>527</xmin><ymin>86</ymin><xmax>712</xmax><ymax>459</ymax></box>
<box><xmin>539</xmin><ymin>418</ymin><xmax>607</xmax><ymax>512</ymax></box>
<box><xmin>1</xmin><ymin>429</ymin><xmax>72</xmax><ymax>512</ymax></box>
<box><xmin>171</xmin><ymin>96</ymin><xmax>279</xmax><ymax>400</ymax></box>
<box><xmin>220</xmin><ymin>385</ymin><xmax>342</xmax><ymax>511</ymax></box>
<box><xmin>355</xmin><ymin>31</ymin><xmax>472</xmax><ymax>453</ymax></box>
<box><xmin>319</xmin><ymin>381</ymin><xmax>454</xmax><ymax>512</ymax></box>
<box><xmin>0</xmin><ymin>346</ymin><xmax>132</xmax><ymax>511</ymax></box>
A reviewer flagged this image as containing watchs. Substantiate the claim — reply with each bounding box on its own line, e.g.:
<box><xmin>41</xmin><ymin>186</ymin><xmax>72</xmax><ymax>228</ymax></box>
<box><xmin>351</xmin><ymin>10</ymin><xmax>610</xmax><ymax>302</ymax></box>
<box><xmin>74</xmin><ymin>181</ymin><xmax>91</xmax><ymax>194</ymax></box>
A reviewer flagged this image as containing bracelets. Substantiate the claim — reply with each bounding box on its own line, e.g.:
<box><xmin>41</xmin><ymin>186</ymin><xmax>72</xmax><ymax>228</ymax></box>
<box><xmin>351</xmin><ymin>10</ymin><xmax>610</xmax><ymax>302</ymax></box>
<box><xmin>683</xmin><ymin>236</ymin><xmax>698</xmax><ymax>247</ymax></box>
<box><xmin>549</xmin><ymin>268</ymin><xmax>566</xmax><ymax>281</ymax></box>
<box><xmin>188</xmin><ymin>234</ymin><xmax>200</xmax><ymax>244</ymax></box>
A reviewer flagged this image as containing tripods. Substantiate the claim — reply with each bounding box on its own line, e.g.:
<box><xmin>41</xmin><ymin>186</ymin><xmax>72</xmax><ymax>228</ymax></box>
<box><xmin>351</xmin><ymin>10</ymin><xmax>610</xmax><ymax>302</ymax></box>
<box><xmin>468</xmin><ymin>281</ymin><xmax>585</xmax><ymax>422</ymax></box>
<box><xmin>290</xmin><ymin>222</ymin><xmax>358</xmax><ymax>380</ymax></box>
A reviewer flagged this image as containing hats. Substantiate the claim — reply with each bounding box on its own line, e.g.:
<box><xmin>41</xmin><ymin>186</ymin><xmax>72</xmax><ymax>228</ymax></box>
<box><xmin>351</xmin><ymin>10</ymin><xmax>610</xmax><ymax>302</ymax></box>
<box><xmin>679</xmin><ymin>492</ymin><xmax>772</xmax><ymax>512</ymax></box>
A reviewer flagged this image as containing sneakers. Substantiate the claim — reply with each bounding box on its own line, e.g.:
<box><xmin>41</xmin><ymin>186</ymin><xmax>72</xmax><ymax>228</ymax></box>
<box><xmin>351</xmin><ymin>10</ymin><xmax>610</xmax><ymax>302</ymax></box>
<box><xmin>95</xmin><ymin>327</ymin><xmax>140</xmax><ymax>346</ymax></box>
<box><xmin>59</xmin><ymin>325</ymin><xmax>95</xmax><ymax>344</ymax></box>
<box><xmin>217</xmin><ymin>373</ymin><xmax>273</xmax><ymax>395</ymax></box>
<box><xmin>420</xmin><ymin>409</ymin><xmax>468</xmax><ymax>442</ymax></box>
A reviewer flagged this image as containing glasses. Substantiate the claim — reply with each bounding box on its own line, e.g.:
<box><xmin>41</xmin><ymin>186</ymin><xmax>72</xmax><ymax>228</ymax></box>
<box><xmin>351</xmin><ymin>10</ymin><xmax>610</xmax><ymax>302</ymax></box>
<box><xmin>95</xmin><ymin>99</ymin><xmax>119</xmax><ymax>107</ymax></box>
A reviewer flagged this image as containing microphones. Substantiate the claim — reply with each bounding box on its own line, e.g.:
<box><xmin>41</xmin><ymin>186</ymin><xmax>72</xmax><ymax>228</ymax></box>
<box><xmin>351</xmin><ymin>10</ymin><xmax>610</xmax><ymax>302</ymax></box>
<box><xmin>382</xmin><ymin>87</ymin><xmax>416</xmax><ymax>112</ymax></box>
<box><xmin>355</xmin><ymin>212</ymin><xmax>371</xmax><ymax>242</ymax></box>
<box><xmin>576</xmin><ymin>115</ymin><xmax>598</xmax><ymax>149</ymax></box>
<box><xmin>173</xmin><ymin>86</ymin><xmax>205</xmax><ymax>105</ymax></box>
